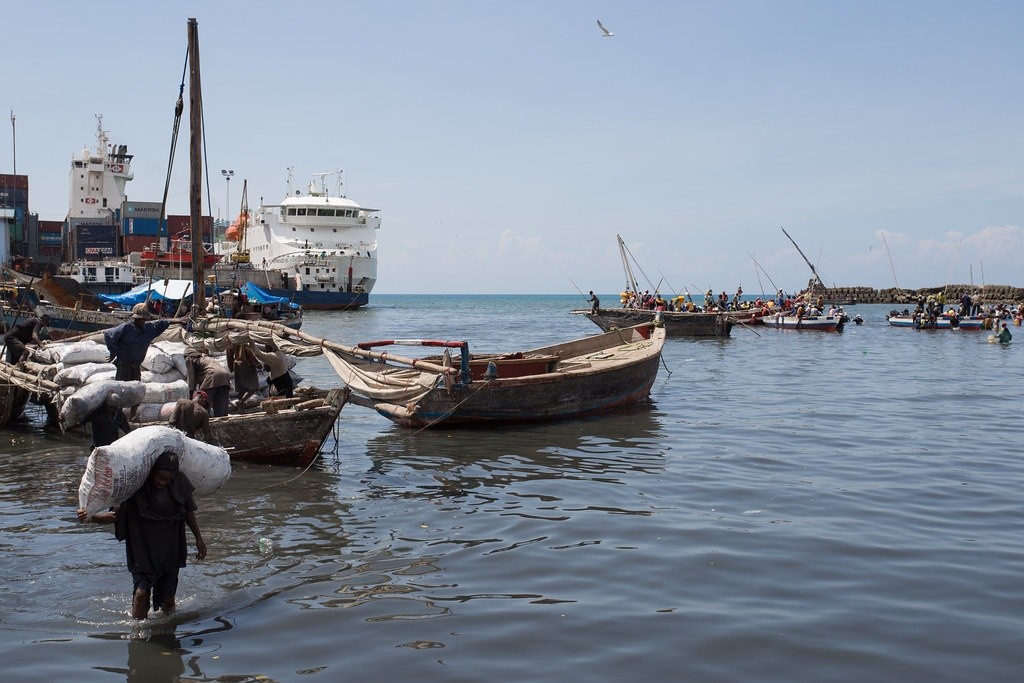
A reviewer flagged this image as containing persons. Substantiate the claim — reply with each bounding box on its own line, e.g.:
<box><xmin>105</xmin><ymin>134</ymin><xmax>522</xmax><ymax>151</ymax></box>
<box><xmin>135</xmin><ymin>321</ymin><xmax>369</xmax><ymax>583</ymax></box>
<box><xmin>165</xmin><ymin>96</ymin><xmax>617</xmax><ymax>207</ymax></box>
<box><xmin>268</xmin><ymin>305</ymin><xmax>278</xmax><ymax>320</ymax></box>
<box><xmin>320</xmin><ymin>252</ymin><xmax>326</xmax><ymax>258</ymax></box>
<box><xmin>59</xmin><ymin>393</ymin><xmax>132</xmax><ymax>458</ymax></box>
<box><xmin>262</xmin><ymin>257</ymin><xmax>266</xmax><ymax>269</ymax></box>
<box><xmin>98</xmin><ymin>250</ymin><xmax>103</xmax><ymax>260</ymax></box>
<box><xmin>852</xmin><ymin>314</ymin><xmax>864</xmax><ymax>327</ymax></box>
<box><xmin>887</xmin><ymin>291</ymin><xmax>1024</xmax><ymax>331</ymax></box>
<box><xmin>747</xmin><ymin>313</ymin><xmax>758</xmax><ymax>325</ymax></box>
<box><xmin>104</xmin><ymin>303</ymin><xmax>187</xmax><ymax>422</ymax></box>
<box><xmin>106</xmin><ymin>298</ymin><xmax>192</xmax><ymax>318</ymax></box>
<box><xmin>183</xmin><ymin>346</ymin><xmax>231</xmax><ymax>418</ymax></box>
<box><xmin>3</xmin><ymin>314</ymin><xmax>50</xmax><ymax>367</ymax></box>
<box><xmin>620</xmin><ymin>287</ymin><xmax>764</xmax><ymax>313</ymax></box>
<box><xmin>168</xmin><ymin>390</ymin><xmax>214</xmax><ymax>445</ymax></box>
<box><xmin>0</xmin><ymin>256</ymin><xmax>57</xmax><ymax>285</ymax></box>
<box><xmin>249</xmin><ymin>337</ymin><xmax>294</xmax><ymax>401</ymax></box>
<box><xmin>229</xmin><ymin>312</ymin><xmax>259</xmax><ymax>395</ymax></box>
<box><xmin>587</xmin><ymin>291</ymin><xmax>600</xmax><ymax>315</ymax></box>
<box><xmin>760</xmin><ymin>289</ymin><xmax>850</xmax><ymax>325</ymax></box>
<box><xmin>994</xmin><ymin>323</ymin><xmax>1012</xmax><ymax>349</ymax></box>
<box><xmin>77</xmin><ymin>448</ymin><xmax>208</xmax><ymax>623</ymax></box>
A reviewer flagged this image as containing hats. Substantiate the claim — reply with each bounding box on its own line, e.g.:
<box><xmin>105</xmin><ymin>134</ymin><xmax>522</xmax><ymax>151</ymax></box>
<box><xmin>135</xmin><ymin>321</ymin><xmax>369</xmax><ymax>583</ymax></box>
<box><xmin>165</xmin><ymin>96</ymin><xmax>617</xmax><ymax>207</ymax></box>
<box><xmin>42</xmin><ymin>314</ymin><xmax>51</xmax><ymax>327</ymax></box>
<box><xmin>131</xmin><ymin>302</ymin><xmax>151</xmax><ymax>319</ymax></box>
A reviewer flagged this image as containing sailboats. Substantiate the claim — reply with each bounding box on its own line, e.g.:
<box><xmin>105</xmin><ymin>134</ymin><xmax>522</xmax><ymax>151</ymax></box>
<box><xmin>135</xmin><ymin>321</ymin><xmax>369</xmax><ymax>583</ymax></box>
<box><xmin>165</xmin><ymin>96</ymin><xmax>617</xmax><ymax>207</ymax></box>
<box><xmin>568</xmin><ymin>225</ymin><xmax>1011</xmax><ymax>338</ymax></box>
<box><xmin>0</xmin><ymin>17</ymin><xmax>351</xmax><ymax>469</ymax></box>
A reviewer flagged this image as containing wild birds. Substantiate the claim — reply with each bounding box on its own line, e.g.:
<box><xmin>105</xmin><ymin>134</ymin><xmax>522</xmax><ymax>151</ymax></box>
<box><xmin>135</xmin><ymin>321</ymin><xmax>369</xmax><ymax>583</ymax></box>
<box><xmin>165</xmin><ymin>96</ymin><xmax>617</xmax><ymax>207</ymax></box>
<box><xmin>870</xmin><ymin>245</ymin><xmax>874</xmax><ymax>249</ymax></box>
<box><xmin>597</xmin><ymin>19</ymin><xmax>616</xmax><ymax>36</ymax></box>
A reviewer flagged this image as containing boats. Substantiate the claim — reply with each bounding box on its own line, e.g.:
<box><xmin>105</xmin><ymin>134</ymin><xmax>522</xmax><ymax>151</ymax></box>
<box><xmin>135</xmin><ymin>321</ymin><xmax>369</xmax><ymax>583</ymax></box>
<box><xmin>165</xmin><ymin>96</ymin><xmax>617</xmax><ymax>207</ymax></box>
<box><xmin>218</xmin><ymin>168</ymin><xmax>383</xmax><ymax>310</ymax></box>
<box><xmin>342</xmin><ymin>305</ymin><xmax>668</xmax><ymax>428</ymax></box>
<box><xmin>226</xmin><ymin>215</ymin><xmax>250</xmax><ymax>240</ymax></box>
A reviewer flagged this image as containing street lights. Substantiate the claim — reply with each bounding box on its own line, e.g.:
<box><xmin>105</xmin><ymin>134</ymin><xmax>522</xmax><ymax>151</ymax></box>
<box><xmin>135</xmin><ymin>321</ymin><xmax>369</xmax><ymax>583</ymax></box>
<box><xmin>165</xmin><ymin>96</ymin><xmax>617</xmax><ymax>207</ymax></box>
<box><xmin>222</xmin><ymin>169</ymin><xmax>235</xmax><ymax>220</ymax></box>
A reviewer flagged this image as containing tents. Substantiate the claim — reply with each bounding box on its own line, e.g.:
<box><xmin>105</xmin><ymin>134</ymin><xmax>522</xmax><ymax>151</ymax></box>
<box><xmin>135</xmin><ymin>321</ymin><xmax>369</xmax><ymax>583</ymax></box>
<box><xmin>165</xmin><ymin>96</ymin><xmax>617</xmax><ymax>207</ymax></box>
<box><xmin>232</xmin><ymin>280</ymin><xmax>298</xmax><ymax>309</ymax></box>
<box><xmin>95</xmin><ymin>278</ymin><xmax>200</xmax><ymax>302</ymax></box>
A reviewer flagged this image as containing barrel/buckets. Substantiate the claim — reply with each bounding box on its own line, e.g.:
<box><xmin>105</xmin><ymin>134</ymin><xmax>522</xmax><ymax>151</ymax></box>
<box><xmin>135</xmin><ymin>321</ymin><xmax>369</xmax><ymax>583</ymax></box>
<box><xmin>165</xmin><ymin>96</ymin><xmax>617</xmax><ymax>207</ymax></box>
<box><xmin>988</xmin><ymin>335</ymin><xmax>995</xmax><ymax>344</ymax></box>
